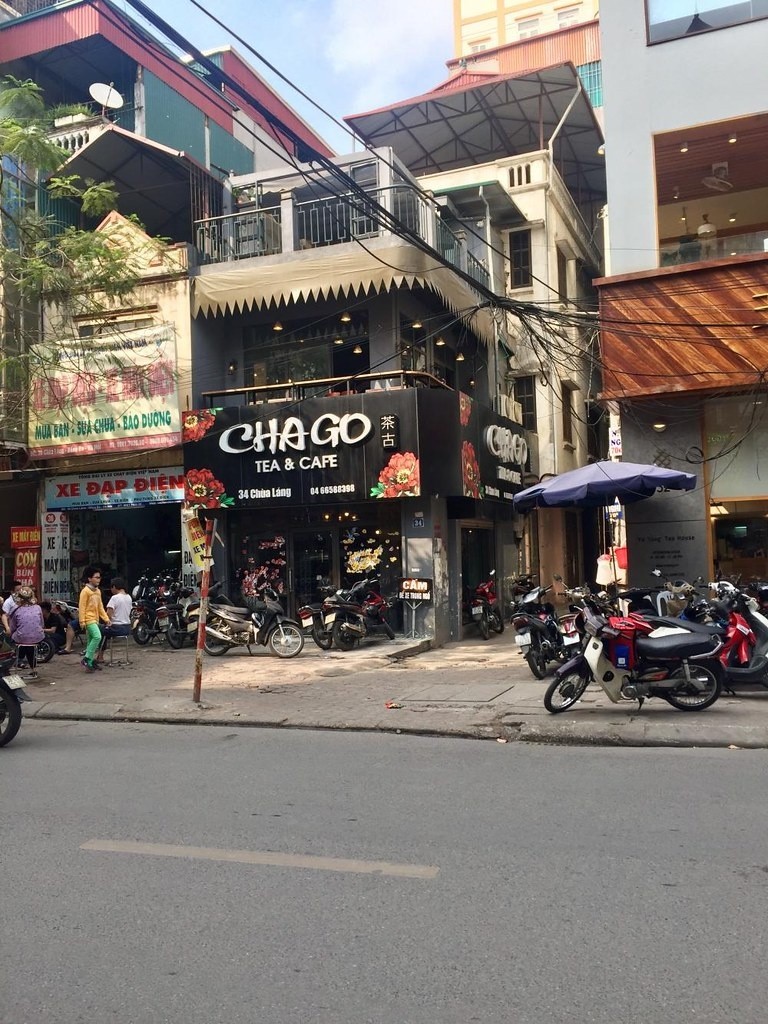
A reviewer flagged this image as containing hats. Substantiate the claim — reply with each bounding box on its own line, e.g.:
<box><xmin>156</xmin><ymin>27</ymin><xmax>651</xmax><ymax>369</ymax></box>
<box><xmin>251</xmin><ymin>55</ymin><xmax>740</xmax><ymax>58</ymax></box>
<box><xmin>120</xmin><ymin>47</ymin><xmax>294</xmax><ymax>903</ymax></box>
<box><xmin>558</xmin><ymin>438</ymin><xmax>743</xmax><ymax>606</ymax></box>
<box><xmin>12</xmin><ymin>587</ymin><xmax>38</xmax><ymax>607</ymax></box>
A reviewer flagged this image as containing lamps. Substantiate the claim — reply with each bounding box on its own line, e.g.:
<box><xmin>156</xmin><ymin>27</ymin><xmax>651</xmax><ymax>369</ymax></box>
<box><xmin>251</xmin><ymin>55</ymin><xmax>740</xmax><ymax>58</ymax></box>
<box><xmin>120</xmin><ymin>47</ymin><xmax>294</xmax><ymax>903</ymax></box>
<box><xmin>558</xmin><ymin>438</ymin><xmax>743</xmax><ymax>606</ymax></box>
<box><xmin>710</xmin><ymin>495</ymin><xmax>729</xmax><ymax>524</ymax></box>
<box><xmin>651</xmin><ymin>411</ymin><xmax>669</xmax><ymax>433</ymax></box>
<box><xmin>470</xmin><ymin>379</ymin><xmax>475</xmax><ymax>386</ymax></box>
<box><xmin>341</xmin><ymin>311</ymin><xmax>351</xmax><ymax>323</ymax></box>
<box><xmin>728</xmin><ymin>133</ymin><xmax>737</xmax><ymax>144</ymax></box>
<box><xmin>515</xmin><ymin>528</ymin><xmax>523</xmax><ymax>548</ymax></box>
<box><xmin>436</xmin><ymin>332</ymin><xmax>446</xmax><ymax>346</ymax></box>
<box><xmin>322</xmin><ymin>510</ymin><xmax>360</xmax><ymax>525</ymax></box>
<box><xmin>334</xmin><ymin>335</ymin><xmax>344</xmax><ymax>345</ymax></box>
<box><xmin>673</xmin><ymin>187</ymin><xmax>745</xmax><ymax>257</ymax></box>
<box><xmin>274</xmin><ymin>318</ymin><xmax>283</xmax><ymax>331</ymax></box>
<box><xmin>598</xmin><ymin>143</ymin><xmax>605</xmax><ymax>155</ymax></box>
<box><xmin>228</xmin><ymin>358</ymin><xmax>239</xmax><ymax>375</ymax></box>
<box><xmin>678</xmin><ymin>141</ymin><xmax>690</xmax><ymax>153</ymax></box>
<box><xmin>354</xmin><ymin>345</ymin><xmax>363</xmax><ymax>355</ymax></box>
<box><xmin>411</xmin><ymin>316</ymin><xmax>422</xmax><ymax>328</ymax></box>
<box><xmin>456</xmin><ymin>351</ymin><xmax>465</xmax><ymax>361</ymax></box>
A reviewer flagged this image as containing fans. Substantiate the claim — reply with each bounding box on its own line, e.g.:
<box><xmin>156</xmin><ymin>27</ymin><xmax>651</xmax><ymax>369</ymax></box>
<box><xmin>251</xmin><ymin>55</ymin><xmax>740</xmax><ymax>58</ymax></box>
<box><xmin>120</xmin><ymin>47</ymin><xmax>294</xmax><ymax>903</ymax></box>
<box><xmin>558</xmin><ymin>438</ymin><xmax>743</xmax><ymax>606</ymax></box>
<box><xmin>701</xmin><ymin>160</ymin><xmax>732</xmax><ymax>193</ymax></box>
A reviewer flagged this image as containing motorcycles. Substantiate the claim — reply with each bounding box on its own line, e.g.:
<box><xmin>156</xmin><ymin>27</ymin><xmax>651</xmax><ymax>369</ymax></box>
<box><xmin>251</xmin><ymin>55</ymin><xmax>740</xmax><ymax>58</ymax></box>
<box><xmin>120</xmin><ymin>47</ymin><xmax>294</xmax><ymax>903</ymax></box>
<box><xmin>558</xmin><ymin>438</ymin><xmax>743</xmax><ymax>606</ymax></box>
<box><xmin>187</xmin><ymin>583</ymin><xmax>304</xmax><ymax>659</ymax></box>
<box><xmin>0</xmin><ymin>596</ymin><xmax>81</xmax><ymax>747</ymax></box>
<box><xmin>509</xmin><ymin>568</ymin><xmax>768</xmax><ymax>714</ymax></box>
<box><xmin>469</xmin><ymin>569</ymin><xmax>503</xmax><ymax>640</ymax></box>
<box><xmin>132</xmin><ymin>563</ymin><xmax>235</xmax><ymax>650</ymax></box>
<box><xmin>296</xmin><ymin>571</ymin><xmax>395</xmax><ymax>651</ymax></box>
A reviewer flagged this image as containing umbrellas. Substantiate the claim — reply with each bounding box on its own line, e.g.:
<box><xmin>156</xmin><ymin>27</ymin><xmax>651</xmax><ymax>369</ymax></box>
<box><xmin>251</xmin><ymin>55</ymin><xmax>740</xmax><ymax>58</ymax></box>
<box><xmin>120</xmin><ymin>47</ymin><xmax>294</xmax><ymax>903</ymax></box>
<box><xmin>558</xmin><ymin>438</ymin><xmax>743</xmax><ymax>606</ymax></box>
<box><xmin>513</xmin><ymin>457</ymin><xmax>697</xmax><ymax>595</ymax></box>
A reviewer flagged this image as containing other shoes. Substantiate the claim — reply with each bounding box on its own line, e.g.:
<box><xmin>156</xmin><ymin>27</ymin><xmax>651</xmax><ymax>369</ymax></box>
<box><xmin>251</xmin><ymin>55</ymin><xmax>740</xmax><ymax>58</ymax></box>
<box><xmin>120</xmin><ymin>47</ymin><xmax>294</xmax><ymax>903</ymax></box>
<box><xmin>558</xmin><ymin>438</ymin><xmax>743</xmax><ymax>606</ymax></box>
<box><xmin>92</xmin><ymin>659</ymin><xmax>103</xmax><ymax>670</ymax></box>
<box><xmin>27</xmin><ymin>671</ymin><xmax>37</xmax><ymax>676</ymax></box>
<box><xmin>13</xmin><ymin>662</ymin><xmax>27</xmax><ymax>669</ymax></box>
<box><xmin>81</xmin><ymin>658</ymin><xmax>94</xmax><ymax>672</ymax></box>
<box><xmin>95</xmin><ymin>659</ymin><xmax>104</xmax><ymax>663</ymax></box>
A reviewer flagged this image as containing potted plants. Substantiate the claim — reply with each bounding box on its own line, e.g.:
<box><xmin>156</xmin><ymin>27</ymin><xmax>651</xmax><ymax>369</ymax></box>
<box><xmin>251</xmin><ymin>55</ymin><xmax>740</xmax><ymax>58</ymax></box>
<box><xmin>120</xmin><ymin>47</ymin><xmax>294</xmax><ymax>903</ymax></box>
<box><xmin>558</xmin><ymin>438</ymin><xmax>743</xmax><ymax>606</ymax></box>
<box><xmin>49</xmin><ymin>103</ymin><xmax>95</xmax><ymax>128</ymax></box>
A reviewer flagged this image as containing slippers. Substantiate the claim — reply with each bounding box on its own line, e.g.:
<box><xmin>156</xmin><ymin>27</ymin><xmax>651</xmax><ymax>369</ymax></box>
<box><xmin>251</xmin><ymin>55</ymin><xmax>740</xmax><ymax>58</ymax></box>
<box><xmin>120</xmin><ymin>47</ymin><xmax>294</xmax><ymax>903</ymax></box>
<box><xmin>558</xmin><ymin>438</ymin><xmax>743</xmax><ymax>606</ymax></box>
<box><xmin>58</xmin><ymin>649</ymin><xmax>70</xmax><ymax>655</ymax></box>
<box><xmin>80</xmin><ymin>650</ymin><xmax>86</xmax><ymax>655</ymax></box>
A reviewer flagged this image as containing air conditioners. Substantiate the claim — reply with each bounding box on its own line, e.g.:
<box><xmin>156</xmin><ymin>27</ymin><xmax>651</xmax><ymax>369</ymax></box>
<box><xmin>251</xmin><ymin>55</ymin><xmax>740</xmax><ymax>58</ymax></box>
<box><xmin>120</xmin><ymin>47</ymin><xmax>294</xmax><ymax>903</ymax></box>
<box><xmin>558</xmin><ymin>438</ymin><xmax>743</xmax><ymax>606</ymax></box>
<box><xmin>493</xmin><ymin>395</ymin><xmax>523</xmax><ymax>426</ymax></box>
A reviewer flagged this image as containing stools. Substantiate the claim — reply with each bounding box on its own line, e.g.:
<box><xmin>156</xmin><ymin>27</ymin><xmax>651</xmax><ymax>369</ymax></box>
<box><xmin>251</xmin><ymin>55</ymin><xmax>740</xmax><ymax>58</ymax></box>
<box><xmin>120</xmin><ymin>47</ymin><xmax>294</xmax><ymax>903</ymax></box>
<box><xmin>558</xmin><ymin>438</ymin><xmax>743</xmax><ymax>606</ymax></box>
<box><xmin>14</xmin><ymin>643</ymin><xmax>38</xmax><ymax>680</ymax></box>
<box><xmin>98</xmin><ymin>635</ymin><xmax>133</xmax><ymax>668</ymax></box>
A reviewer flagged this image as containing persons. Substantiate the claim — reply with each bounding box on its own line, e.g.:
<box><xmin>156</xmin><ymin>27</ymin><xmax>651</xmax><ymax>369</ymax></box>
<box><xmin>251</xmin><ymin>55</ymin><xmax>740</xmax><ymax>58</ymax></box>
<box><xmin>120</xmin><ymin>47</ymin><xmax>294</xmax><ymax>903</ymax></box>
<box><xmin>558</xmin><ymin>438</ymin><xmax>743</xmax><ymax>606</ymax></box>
<box><xmin>59</xmin><ymin>619</ymin><xmax>80</xmax><ymax>655</ymax></box>
<box><xmin>1</xmin><ymin>581</ymin><xmax>22</xmax><ymax>636</ymax></box>
<box><xmin>96</xmin><ymin>578</ymin><xmax>132</xmax><ymax>663</ymax></box>
<box><xmin>78</xmin><ymin>567</ymin><xmax>112</xmax><ymax>671</ymax></box>
<box><xmin>9</xmin><ymin>587</ymin><xmax>44</xmax><ymax>674</ymax></box>
<box><xmin>40</xmin><ymin>602</ymin><xmax>66</xmax><ymax>653</ymax></box>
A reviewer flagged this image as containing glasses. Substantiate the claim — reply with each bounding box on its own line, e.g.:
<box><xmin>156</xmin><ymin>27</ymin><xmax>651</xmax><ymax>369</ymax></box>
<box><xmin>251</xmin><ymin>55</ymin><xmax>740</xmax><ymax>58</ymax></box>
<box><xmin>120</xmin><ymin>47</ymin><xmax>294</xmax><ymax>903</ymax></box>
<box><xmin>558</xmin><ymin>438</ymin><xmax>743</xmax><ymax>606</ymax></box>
<box><xmin>91</xmin><ymin>577</ymin><xmax>101</xmax><ymax>579</ymax></box>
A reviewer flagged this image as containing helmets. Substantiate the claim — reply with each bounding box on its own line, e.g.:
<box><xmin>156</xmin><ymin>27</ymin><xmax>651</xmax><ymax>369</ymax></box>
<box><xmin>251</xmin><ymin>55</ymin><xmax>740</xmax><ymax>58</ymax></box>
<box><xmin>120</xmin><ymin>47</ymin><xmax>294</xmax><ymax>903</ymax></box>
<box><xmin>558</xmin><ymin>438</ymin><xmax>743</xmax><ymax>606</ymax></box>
<box><xmin>366</xmin><ymin>598</ymin><xmax>381</xmax><ymax>615</ymax></box>
